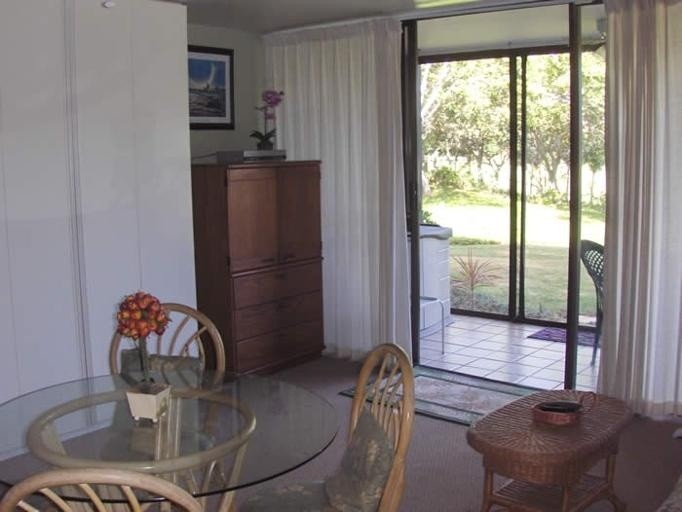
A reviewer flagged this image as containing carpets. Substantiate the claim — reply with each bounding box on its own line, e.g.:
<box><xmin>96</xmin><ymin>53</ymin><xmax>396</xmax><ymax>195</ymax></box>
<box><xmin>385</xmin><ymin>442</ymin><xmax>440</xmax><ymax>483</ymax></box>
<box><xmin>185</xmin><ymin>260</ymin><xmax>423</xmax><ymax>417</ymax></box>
<box><xmin>526</xmin><ymin>326</ymin><xmax>600</xmax><ymax>346</ymax></box>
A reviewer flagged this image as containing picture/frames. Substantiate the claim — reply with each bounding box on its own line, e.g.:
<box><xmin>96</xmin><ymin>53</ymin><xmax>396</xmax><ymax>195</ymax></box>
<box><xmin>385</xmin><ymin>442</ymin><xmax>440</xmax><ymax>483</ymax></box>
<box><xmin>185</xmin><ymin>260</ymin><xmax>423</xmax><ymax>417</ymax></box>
<box><xmin>188</xmin><ymin>45</ymin><xmax>234</xmax><ymax>130</ymax></box>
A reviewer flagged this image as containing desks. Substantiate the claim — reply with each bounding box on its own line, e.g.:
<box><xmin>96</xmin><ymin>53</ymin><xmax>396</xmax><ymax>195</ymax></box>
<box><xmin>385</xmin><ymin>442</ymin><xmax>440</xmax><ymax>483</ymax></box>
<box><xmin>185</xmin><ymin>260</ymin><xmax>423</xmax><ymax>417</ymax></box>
<box><xmin>465</xmin><ymin>389</ymin><xmax>631</xmax><ymax>510</ymax></box>
<box><xmin>0</xmin><ymin>365</ymin><xmax>345</xmax><ymax>510</ymax></box>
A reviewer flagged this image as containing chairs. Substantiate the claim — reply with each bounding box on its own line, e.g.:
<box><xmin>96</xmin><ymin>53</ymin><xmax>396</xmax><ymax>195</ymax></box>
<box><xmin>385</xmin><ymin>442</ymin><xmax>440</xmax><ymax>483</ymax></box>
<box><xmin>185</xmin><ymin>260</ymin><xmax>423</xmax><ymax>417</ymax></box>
<box><xmin>106</xmin><ymin>304</ymin><xmax>229</xmax><ymax>495</ymax></box>
<box><xmin>1</xmin><ymin>465</ymin><xmax>203</xmax><ymax>510</ymax></box>
<box><xmin>236</xmin><ymin>342</ymin><xmax>414</xmax><ymax>508</ymax></box>
<box><xmin>579</xmin><ymin>241</ymin><xmax>607</xmax><ymax>365</ymax></box>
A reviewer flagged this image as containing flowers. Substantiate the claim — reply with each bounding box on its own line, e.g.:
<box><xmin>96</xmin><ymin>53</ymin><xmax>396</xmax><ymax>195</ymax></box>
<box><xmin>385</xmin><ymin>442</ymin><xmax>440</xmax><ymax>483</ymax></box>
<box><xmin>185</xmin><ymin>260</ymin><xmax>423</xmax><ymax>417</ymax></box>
<box><xmin>248</xmin><ymin>91</ymin><xmax>285</xmax><ymax>140</ymax></box>
<box><xmin>118</xmin><ymin>291</ymin><xmax>174</xmax><ymax>390</ymax></box>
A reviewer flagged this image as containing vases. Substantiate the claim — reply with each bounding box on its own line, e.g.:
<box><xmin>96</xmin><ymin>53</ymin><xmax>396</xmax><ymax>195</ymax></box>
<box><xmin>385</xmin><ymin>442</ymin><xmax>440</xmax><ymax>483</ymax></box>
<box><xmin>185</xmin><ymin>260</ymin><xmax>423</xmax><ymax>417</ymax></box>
<box><xmin>126</xmin><ymin>380</ymin><xmax>174</xmax><ymax>424</ymax></box>
<box><xmin>257</xmin><ymin>141</ymin><xmax>273</xmax><ymax>151</ymax></box>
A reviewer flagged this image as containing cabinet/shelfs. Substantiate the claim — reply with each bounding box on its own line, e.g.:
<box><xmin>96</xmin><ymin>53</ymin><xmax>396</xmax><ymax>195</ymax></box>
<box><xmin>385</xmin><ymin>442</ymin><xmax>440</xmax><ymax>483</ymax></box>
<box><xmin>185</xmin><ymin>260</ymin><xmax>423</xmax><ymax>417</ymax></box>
<box><xmin>192</xmin><ymin>160</ymin><xmax>328</xmax><ymax>381</ymax></box>
